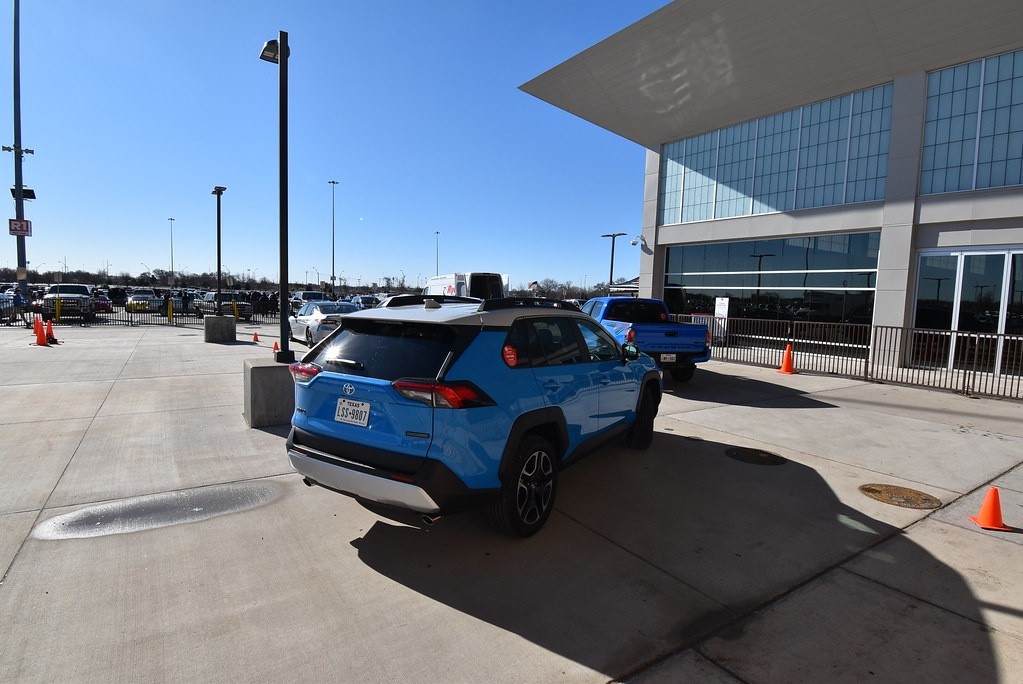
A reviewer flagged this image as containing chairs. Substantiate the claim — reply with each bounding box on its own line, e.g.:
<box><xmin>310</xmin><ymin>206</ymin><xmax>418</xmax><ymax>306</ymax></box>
<box><xmin>529</xmin><ymin>328</ymin><xmax>553</xmax><ymax>358</ymax></box>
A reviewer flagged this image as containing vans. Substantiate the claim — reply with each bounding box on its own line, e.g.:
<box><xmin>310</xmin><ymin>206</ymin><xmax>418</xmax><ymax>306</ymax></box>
<box><xmin>420</xmin><ymin>273</ymin><xmax>504</xmax><ymax>301</ymax></box>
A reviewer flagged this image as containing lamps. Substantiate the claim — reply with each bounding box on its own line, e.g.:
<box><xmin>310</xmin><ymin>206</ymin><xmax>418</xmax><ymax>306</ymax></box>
<box><xmin>631</xmin><ymin>235</ymin><xmax>646</xmax><ymax>246</ymax></box>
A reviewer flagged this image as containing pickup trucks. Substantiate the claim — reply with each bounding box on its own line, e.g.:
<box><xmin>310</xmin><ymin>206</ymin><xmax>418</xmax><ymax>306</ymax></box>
<box><xmin>193</xmin><ymin>292</ymin><xmax>252</xmax><ymax>321</ymax></box>
<box><xmin>576</xmin><ymin>295</ymin><xmax>712</xmax><ymax>382</ymax></box>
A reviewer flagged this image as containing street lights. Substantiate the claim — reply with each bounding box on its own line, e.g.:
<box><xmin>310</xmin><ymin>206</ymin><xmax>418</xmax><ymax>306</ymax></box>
<box><xmin>168</xmin><ymin>217</ymin><xmax>175</xmax><ymax>289</ymax></box>
<box><xmin>259</xmin><ymin>31</ymin><xmax>295</xmax><ymax>362</ymax></box>
<box><xmin>211</xmin><ymin>186</ymin><xmax>227</xmax><ymax>316</ymax></box>
<box><xmin>329</xmin><ymin>180</ymin><xmax>339</xmax><ymax>289</ymax></box>
<box><xmin>601</xmin><ymin>233</ymin><xmax>627</xmax><ymax>285</ymax></box>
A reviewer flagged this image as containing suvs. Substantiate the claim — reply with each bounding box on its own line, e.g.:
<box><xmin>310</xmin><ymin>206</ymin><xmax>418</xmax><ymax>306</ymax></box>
<box><xmin>285</xmin><ymin>295</ymin><xmax>662</xmax><ymax>535</ymax></box>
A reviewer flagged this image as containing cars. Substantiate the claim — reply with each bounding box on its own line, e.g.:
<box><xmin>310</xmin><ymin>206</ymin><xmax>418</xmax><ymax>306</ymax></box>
<box><xmin>126</xmin><ymin>289</ymin><xmax>205</xmax><ymax>317</ymax></box>
<box><xmin>562</xmin><ymin>299</ymin><xmax>587</xmax><ymax>309</ymax></box>
<box><xmin>336</xmin><ymin>293</ymin><xmax>484</xmax><ymax>308</ymax></box>
<box><xmin>0</xmin><ymin>284</ymin><xmax>113</xmax><ymax>320</ymax></box>
<box><xmin>288</xmin><ymin>291</ymin><xmax>333</xmax><ymax>308</ymax></box>
<box><xmin>287</xmin><ymin>302</ymin><xmax>361</xmax><ymax>348</ymax></box>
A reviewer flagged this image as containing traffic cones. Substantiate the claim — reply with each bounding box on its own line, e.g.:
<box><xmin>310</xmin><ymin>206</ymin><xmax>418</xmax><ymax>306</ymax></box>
<box><xmin>967</xmin><ymin>487</ymin><xmax>1014</xmax><ymax>533</ymax></box>
<box><xmin>32</xmin><ymin>316</ymin><xmax>54</xmax><ymax>346</ymax></box>
<box><xmin>272</xmin><ymin>342</ymin><xmax>280</xmax><ymax>353</ymax></box>
<box><xmin>777</xmin><ymin>343</ymin><xmax>799</xmax><ymax>375</ymax></box>
<box><xmin>254</xmin><ymin>332</ymin><xmax>259</xmax><ymax>341</ymax></box>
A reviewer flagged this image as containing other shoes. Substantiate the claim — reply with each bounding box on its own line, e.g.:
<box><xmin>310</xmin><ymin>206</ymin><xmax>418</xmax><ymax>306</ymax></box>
<box><xmin>26</xmin><ymin>321</ymin><xmax>33</xmax><ymax>325</ymax></box>
<box><xmin>7</xmin><ymin>323</ymin><xmax>14</xmax><ymax>326</ymax></box>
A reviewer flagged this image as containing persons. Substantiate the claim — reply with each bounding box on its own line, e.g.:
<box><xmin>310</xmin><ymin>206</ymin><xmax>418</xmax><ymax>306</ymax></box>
<box><xmin>182</xmin><ymin>292</ymin><xmax>189</xmax><ymax>315</ymax></box>
<box><xmin>332</xmin><ymin>292</ymin><xmax>357</xmax><ymax>301</ymax></box>
<box><xmin>163</xmin><ymin>291</ymin><xmax>170</xmax><ymax>316</ymax></box>
<box><xmin>241</xmin><ymin>291</ymin><xmax>277</xmax><ymax>316</ymax></box>
<box><xmin>6</xmin><ymin>288</ymin><xmax>28</xmax><ymax>326</ymax></box>
